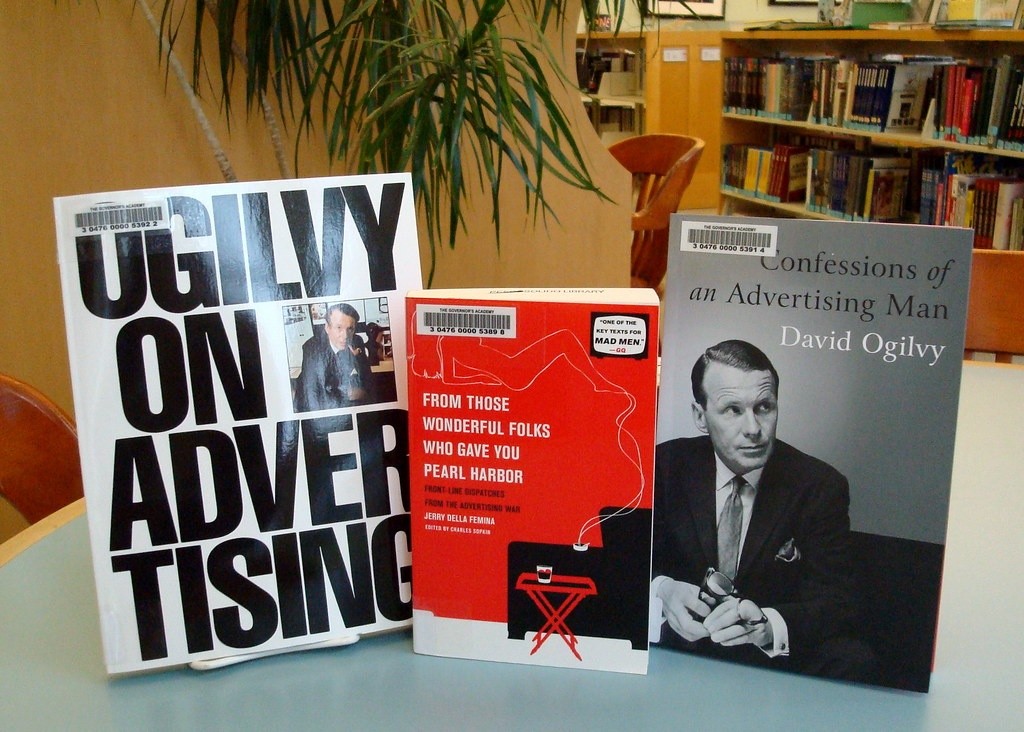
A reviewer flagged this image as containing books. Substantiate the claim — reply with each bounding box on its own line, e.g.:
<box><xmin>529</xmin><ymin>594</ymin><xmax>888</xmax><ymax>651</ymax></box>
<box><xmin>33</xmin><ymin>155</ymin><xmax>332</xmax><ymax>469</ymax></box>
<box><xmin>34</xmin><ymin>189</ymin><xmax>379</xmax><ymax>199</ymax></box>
<box><xmin>574</xmin><ymin>45</ymin><xmax>635</xmax><ymax>133</ymax></box>
<box><xmin>743</xmin><ymin>18</ymin><xmax>1013</xmax><ymax>32</ymax></box>
<box><xmin>721</xmin><ymin>54</ymin><xmax>1024</xmax><ymax>252</ymax></box>
<box><xmin>649</xmin><ymin>213</ymin><xmax>974</xmax><ymax>697</ymax></box>
<box><xmin>404</xmin><ymin>286</ymin><xmax>659</xmax><ymax>675</ymax></box>
<box><xmin>54</xmin><ymin>172</ymin><xmax>423</xmax><ymax>677</ymax></box>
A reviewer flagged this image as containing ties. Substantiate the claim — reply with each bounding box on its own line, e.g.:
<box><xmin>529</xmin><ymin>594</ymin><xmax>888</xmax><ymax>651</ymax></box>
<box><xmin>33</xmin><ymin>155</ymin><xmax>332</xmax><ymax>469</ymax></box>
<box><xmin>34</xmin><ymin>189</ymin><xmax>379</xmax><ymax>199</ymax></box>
<box><xmin>718</xmin><ymin>474</ymin><xmax>746</xmax><ymax>582</ymax></box>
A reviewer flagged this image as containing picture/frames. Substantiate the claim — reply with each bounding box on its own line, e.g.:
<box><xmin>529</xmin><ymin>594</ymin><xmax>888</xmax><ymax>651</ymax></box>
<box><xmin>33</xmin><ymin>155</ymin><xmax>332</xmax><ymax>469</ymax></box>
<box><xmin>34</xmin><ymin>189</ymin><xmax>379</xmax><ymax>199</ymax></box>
<box><xmin>643</xmin><ymin>0</ymin><xmax>726</xmax><ymax>20</ymax></box>
<box><xmin>768</xmin><ymin>0</ymin><xmax>841</xmax><ymax>6</ymax></box>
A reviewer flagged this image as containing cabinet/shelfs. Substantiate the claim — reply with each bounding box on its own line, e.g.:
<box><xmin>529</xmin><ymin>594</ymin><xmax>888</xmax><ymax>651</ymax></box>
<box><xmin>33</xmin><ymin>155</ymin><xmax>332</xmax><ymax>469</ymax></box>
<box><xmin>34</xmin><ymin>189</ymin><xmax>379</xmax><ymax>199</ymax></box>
<box><xmin>718</xmin><ymin>28</ymin><xmax>1024</xmax><ymax>251</ymax></box>
<box><xmin>574</xmin><ymin>32</ymin><xmax>721</xmax><ymax>210</ymax></box>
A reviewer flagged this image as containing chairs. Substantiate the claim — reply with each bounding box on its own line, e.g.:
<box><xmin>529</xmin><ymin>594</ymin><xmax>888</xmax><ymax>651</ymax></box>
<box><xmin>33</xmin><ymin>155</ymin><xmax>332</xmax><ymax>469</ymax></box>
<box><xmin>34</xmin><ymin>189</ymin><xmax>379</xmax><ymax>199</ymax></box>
<box><xmin>607</xmin><ymin>133</ymin><xmax>705</xmax><ymax>289</ymax></box>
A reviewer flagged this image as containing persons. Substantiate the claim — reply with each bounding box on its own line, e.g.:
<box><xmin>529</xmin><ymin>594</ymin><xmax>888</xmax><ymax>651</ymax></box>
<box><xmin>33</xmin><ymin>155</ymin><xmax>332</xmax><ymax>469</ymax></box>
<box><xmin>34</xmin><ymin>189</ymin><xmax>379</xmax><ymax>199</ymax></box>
<box><xmin>294</xmin><ymin>303</ymin><xmax>375</xmax><ymax>412</ymax></box>
<box><xmin>657</xmin><ymin>340</ymin><xmax>853</xmax><ymax>658</ymax></box>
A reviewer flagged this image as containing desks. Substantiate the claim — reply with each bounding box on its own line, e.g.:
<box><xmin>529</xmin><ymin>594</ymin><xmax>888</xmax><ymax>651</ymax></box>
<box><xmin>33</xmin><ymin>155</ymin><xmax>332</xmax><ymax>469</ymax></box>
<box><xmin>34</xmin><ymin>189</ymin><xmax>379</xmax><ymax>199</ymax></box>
<box><xmin>0</xmin><ymin>360</ymin><xmax>1024</xmax><ymax>730</ymax></box>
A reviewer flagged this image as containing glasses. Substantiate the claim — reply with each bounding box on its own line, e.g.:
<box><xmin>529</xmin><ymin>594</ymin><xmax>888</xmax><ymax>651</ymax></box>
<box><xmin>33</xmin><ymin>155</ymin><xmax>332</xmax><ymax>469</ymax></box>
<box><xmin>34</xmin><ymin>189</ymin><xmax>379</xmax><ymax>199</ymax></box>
<box><xmin>699</xmin><ymin>567</ymin><xmax>768</xmax><ymax>626</ymax></box>
